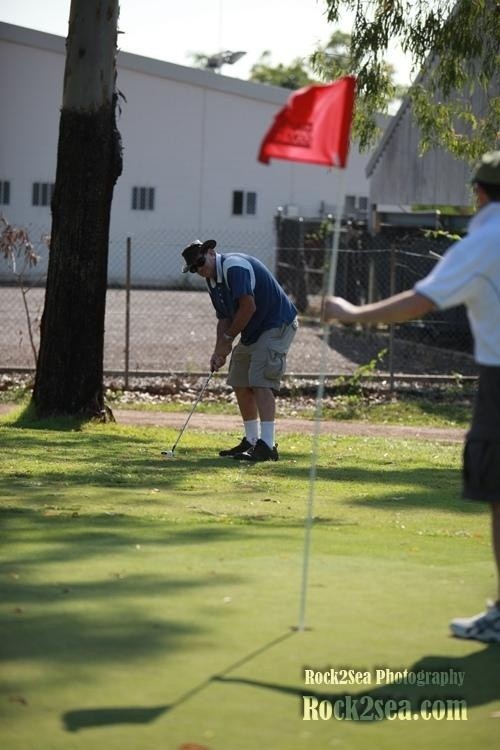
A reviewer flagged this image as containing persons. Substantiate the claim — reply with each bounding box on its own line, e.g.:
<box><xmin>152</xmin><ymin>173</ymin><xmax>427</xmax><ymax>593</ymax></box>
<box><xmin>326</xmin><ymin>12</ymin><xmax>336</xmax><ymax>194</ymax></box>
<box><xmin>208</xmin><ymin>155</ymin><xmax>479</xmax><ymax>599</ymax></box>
<box><xmin>318</xmin><ymin>149</ymin><xmax>498</xmax><ymax>650</ymax></box>
<box><xmin>180</xmin><ymin>237</ymin><xmax>298</xmax><ymax>463</ymax></box>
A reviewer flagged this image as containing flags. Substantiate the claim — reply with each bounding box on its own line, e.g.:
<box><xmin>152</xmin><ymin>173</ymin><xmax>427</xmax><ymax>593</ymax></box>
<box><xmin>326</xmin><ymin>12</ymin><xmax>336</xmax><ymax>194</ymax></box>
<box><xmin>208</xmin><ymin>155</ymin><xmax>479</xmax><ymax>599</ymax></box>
<box><xmin>255</xmin><ymin>75</ymin><xmax>355</xmax><ymax>170</ymax></box>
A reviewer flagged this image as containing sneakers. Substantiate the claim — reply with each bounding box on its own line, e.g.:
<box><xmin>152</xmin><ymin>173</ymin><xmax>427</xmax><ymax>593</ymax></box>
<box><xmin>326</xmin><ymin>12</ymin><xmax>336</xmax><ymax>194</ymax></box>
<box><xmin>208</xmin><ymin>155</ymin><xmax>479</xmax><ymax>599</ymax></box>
<box><xmin>219</xmin><ymin>437</ymin><xmax>253</xmax><ymax>456</ymax></box>
<box><xmin>234</xmin><ymin>439</ymin><xmax>278</xmax><ymax>461</ymax></box>
<box><xmin>450</xmin><ymin>601</ymin><xmax>500</xmax><ymax>642</ymax></box>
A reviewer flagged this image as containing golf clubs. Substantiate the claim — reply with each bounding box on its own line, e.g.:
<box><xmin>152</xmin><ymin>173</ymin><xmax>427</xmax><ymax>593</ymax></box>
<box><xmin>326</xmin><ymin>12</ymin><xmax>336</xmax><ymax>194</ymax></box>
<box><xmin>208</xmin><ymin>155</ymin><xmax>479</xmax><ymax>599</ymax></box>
<box><xmin>161</xmin><ymin>370</ymin><xmax>212</xmax><ymax>457</ymax></box>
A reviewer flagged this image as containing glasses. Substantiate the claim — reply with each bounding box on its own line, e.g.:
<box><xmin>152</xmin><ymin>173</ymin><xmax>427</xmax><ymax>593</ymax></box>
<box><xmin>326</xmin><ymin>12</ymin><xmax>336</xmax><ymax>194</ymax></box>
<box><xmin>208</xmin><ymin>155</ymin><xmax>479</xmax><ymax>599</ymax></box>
<box><xmin>189</xmin><ymin>250</ymin><xmax>206</xmax><ymax>273</ymax></box>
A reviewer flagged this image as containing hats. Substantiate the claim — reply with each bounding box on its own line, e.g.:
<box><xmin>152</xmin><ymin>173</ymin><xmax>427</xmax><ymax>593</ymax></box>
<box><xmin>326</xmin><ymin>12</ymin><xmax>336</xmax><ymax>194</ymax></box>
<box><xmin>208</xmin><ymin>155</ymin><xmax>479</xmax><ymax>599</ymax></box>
<box><xmin>181</xmin><ymin>239</ymin><xmax>216</xmax><ymax>273</ymax></box>
<box><xmin>473</xmin><ymin>150</ymin><xmax>500</xmax><ymax>185</ymax></box>
